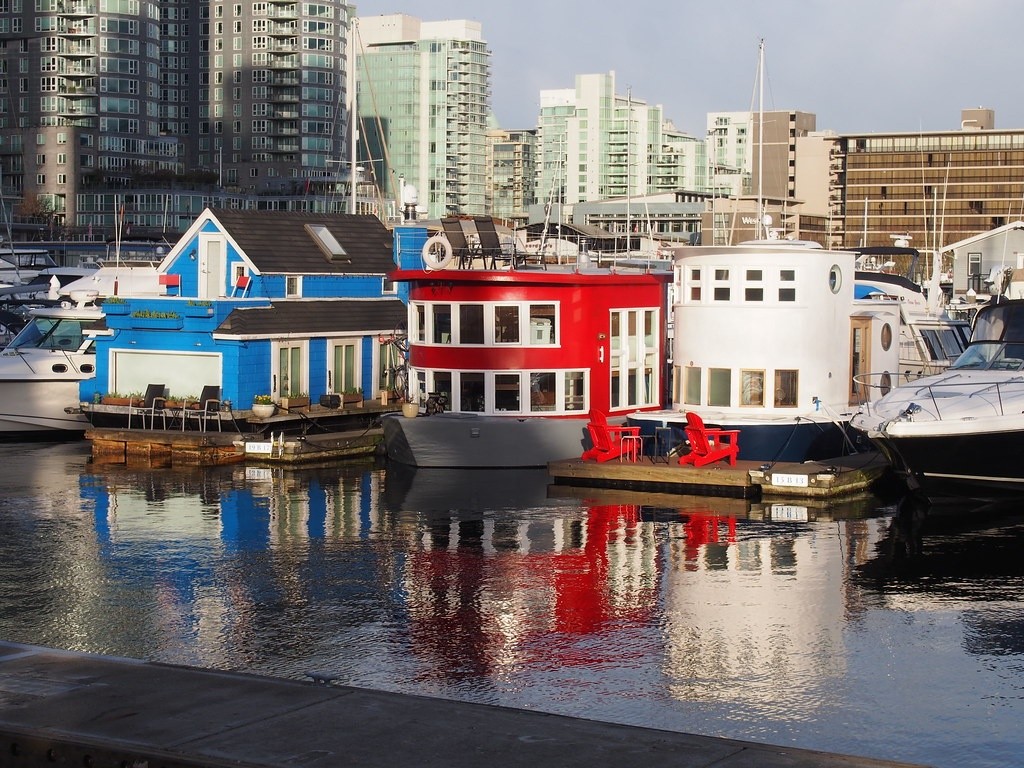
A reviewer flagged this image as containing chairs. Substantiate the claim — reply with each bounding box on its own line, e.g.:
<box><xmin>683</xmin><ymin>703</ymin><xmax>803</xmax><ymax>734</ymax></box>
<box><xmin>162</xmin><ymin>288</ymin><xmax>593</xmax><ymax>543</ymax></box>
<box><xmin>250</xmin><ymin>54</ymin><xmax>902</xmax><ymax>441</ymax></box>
<box><xmin>159</xmin><ymin>275</ymin><xmax>181</xmax><ymax>297</ymax></box>
<box><xmin>231</xmin><ymin>275</ymin><xmax>251</xmax><ymax>298</ymax></box>
<box><xmin>129</xmin><ymin>384</ymin><xmax>166</xmax><ymax>431</ymax></box>
<box><xmin>440</xmin><ymin>217</ymin><xmax>546</xmax><ymax>270</ymax></box>
<box><xmin>679</xmin><ymin>413</ymin><xmax>741</xmax><ymax>467</ymax></box>
<box><xmin>582</xmin><ymin>409</ymin><xmax>641</xmax><ymax>464</ymax></box>
<box><xmin>184</xmin><ymin>386</ymin><xmax>222</xmax><ymax>433</ymax></box>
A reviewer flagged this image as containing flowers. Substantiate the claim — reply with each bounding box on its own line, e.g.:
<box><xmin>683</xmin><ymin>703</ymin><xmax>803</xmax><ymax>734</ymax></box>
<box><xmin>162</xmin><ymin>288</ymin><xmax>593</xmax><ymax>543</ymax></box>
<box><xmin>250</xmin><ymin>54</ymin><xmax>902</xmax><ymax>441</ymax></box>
<box><xmin>255</xmin><ymin>395</ymin><xmax>272</xmax><ymax>405</ymax></box>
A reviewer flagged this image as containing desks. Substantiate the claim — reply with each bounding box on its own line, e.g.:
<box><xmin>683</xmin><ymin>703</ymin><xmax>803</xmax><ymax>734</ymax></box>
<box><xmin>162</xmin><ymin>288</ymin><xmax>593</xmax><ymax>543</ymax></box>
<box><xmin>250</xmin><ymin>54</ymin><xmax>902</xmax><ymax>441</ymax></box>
<box><xmin>655</xmin><ymin>427</ymin><xmax>672</xmax><ymax>465</ymax></box>
<box><xmin>167</xmin><ymin>407</ymin><xmax>192</xmax><ymax>432</ymax></box>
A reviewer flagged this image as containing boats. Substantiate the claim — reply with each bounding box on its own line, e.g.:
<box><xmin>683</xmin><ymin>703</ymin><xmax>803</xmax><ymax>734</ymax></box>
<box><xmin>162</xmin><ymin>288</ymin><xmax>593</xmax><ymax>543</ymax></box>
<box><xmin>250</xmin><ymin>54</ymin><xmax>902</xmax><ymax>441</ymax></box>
<box><xmin>847</xmin><ymin>199</ymin><xmax>1023</xmax><ymax>505</ymax></box>
<box><xmin>0</xmin><ymin>191</ymin><xmax>165</xmax><ymax>434</ymax></box>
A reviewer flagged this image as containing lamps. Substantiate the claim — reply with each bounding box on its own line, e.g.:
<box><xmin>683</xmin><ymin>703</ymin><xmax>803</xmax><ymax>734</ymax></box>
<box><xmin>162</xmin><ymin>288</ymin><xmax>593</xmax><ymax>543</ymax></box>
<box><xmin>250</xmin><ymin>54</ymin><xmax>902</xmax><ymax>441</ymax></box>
<box><xmin>189</xmin><ymin>250</ymin><xmax>196</xmax><ymax>261</ymax></box>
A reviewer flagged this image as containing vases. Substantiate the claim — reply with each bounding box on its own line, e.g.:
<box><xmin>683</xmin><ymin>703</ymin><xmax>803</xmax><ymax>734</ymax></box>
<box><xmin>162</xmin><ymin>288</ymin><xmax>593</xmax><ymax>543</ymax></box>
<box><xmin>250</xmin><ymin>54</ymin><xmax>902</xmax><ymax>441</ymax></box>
<box><xmin>252</xmin><ymin>404</ymin><xmax>275</xmax><ymax>418</ymax></box>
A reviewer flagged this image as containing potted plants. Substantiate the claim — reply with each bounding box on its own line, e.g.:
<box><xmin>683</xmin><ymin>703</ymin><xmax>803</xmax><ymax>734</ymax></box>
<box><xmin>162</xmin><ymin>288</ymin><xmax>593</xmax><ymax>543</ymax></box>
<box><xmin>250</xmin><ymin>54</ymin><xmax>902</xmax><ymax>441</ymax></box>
<box><xmin>185</xmin><ymin>300</ymin><xmax>213</xmax><ymax>317</ymax></box>
<box><xmin>381</xmin><ymin>386</ymin><xmax>402</xmax><ymax>405</ymax></box>
<box><xmin>103</xmin><ymin>390</ymin><xmax>144</xmax><ymax>406</ymax></box>
<box><xmin>164</xmin><ymin>396</ymin><xmax>200</xmax><ymax>408</ymax></box>
<box><xmin>338</xmin><ymin>387</ymin><xmax>364</xmax><ymax>409</ymax></box>
<box><xmin>402</xmin><ymin>394</ymin><xmax>419</xmax><ymax>417</ymax></box>
<box><xmin>101</xmin><ymin>297</ymin><xmax>131</xmax><ymax>315</ymax></box>
<box><xmin>131</xmin><ymin>308</ymin><xmax>183</xmax><ymax>329</ymax></box>
<box><xmin>282</xmin><ymin>391</ymin><xmax>310</xmax><ymax>414</ymax></box>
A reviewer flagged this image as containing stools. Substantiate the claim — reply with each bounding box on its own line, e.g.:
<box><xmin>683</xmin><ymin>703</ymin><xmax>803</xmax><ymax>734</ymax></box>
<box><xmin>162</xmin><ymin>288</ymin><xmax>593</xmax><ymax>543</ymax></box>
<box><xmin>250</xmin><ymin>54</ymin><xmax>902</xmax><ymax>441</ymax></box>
<box><xmin>620</xmin><ymin>436</ymin><xmax>643</xmax><ymax>464</ymax></box>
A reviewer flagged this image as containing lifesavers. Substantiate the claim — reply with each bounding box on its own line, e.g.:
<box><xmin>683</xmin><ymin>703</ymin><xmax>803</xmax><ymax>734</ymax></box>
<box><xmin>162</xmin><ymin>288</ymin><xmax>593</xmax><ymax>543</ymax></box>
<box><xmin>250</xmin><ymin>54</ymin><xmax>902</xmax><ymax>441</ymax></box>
<box><xmin>422</xmin><ymin>235</ymin><xmax>452</xmax><ymax>269</ymax></box>
